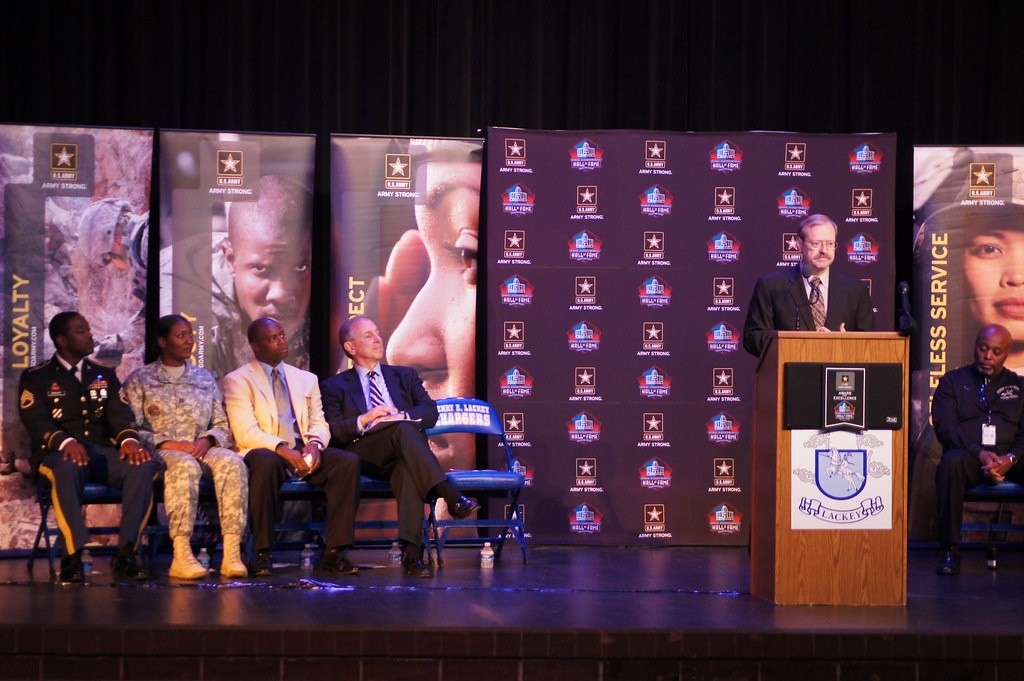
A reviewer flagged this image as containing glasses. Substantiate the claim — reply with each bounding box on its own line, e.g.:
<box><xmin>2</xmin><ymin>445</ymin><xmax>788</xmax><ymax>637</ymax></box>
<box><xmin>804</xmin><ymin>239</ymin><xmax>838</xmax><ymax>249</ymax></box>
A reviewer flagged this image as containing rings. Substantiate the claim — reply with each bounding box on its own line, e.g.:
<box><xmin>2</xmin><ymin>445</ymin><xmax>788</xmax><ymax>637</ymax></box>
<box><xmin>138</xmin><ymin>448</ymin><xmax>143</xmax><ymax>451</ymax></box>
<box><xmin>199</xmin><ymin>456</ymin><xmax>202</xmax><ymax>461</ymax></box>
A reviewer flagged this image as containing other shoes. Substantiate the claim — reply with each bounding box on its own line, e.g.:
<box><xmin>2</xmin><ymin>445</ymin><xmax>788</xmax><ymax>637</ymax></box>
<box><xmin>314</xmin><ymin>557</ymin><xmax>359</xmax><ymax>575</ymax></box>
<box><xmin>109</xmin><ymin>552</ymin><xmax>140</xmax><ymax>575</ymax></box>
<box><xmin>59</xmin><ymin>555</ymin><xmax>86</xmax><ymax>588</ymax></box>
<box><xmin>255</xmin><ymin>554</ymin><xmax>276</xmax><ymax>576</ymax></box>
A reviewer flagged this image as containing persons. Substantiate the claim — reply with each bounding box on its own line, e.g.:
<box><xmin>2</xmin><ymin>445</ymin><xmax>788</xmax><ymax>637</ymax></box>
<box><xmin>914</xmin><ymin>152</ymin><xmax>1024</xmax><ymax>374</ymax></box>
<box><xmin>80</xmin><ymin>197</ymin><xmax>149</xmax><ymax>371</ymax></box>
<box><xmin>743</xmin><ymin>214</ymin><xmax>874</xmax><ymax>357</ymax></box>
<box><xmin>123</xmin><ymin>314</ymin><xmax>247</xmax><ymax>578</ymax></box>
<box><xmin>214</xmin><ymin>173</ymin><xmax>310</xmax><ymax>371</ymax></box>
<box><xmin>330</xmin><ymin>131</ymin><xmax>481</xmax><ymax>536</ymax></box>
<box><xmin>931</xmin><ymin>324</ymin><xmax>1024</xmax><ymax>574</ymax></box>
<box><xmin>223</xmin><ymin>318</ymin><xmax>361</xmax><ymax>574</ymax></box>
<box><xmin>324</xmin><ymin>317</ymin><xmax>481</xmax><ymax>577</ymax></box>
<box><xmin>18</xmin><ymin>311</ymin><xmax>158</xmax><ymax>580</ymax></box>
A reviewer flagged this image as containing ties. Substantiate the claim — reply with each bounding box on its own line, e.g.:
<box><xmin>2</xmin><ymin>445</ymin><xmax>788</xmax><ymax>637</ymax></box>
<box><xmin>273</xmin><ymin>370</ymin><xmax>296</xmax><ymax>450</ymax></box>
<box><xmin>368</xmin><ymin>372</ymin><xmax>386</xmax><ymax>408</ymax></box>
<box><xmin>69</xmin><ymin>367</ymin><xmax>80</xmax><ymax>387</ymax></box>
<box><xmin>810</xmin><ymin>277</ymin><xmax>826</xmax><ymax>330</ymax></box>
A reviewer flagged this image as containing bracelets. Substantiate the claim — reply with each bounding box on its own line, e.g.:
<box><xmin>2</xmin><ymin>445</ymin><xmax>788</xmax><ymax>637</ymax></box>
<box><xmin>311</xmin><ymin>441</ymin><xmax>322</xmax><ymax>451</ymax></box>
<box><xmin>399</xmin><ymin>410</ymin><xmax>407</xmax><ymax>419</ymax></box>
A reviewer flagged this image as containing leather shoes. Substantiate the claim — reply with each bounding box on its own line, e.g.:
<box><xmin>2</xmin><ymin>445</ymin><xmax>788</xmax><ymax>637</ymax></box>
<box><xmin>936</xmin><ymin>551</ymin><xmax>960</xmax><ymax>578</ymax></box>
<box><xmin>406</xmin><ymin>558</ymin><xmax>432</xmax><ymax>578</ymax></box>
<box><xmin>447</xmin><ymin>495</ymin><xmax>481</xmax><ymax>521</ymax></box>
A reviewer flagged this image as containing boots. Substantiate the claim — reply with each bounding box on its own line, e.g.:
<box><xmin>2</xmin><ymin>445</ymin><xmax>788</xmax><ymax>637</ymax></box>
<box><xmin>221</xmin><ymin>535</ymin><xmax>248</xmax><ymax>578</ymax></box>
<box><xmin>169</xmin><ymin>536</ymin><xmax>208</xmax><ymax>579</ymax></box>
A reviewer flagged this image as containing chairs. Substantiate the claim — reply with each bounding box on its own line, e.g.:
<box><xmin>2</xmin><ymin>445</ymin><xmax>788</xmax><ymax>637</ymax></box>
<box><xmin>25</xmin><ymin>398</ymin><xmax>532</xmax><ymax>579</ymax></box>
<box><xmin>960</xmin><ymin>477</ymin><xmax>1024</xmax><ymax>552</ymax></box>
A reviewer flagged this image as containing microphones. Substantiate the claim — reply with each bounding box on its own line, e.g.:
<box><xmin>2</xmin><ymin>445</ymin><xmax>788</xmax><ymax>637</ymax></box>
<box><xmin>897</xmin><ymin>281</ymin><xmax>917</xmax><ymax>333</ymax></box>
<box><xmin>795</xmin><ymin>264</ymin><xmax>819</xmax><ymax>330</ymax></box>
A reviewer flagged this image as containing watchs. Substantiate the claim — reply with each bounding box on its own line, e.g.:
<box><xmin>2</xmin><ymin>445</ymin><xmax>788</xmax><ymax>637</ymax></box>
<box><xmin>1007</xmin><ymin>453</ymin><xmax>1017</xmax><ymax>464</ymax></box>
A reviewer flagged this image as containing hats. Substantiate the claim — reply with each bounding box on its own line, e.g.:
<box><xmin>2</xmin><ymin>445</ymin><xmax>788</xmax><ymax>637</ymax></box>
<box><xmin>918</xmin><ymin>156</ymin><xmax>1024</xmax><ymax>250</ymax></box>
<box><xmin>79</xmin><ymin>198</ymin><xmax>134</xmax><ymax>270</ymax></box>
